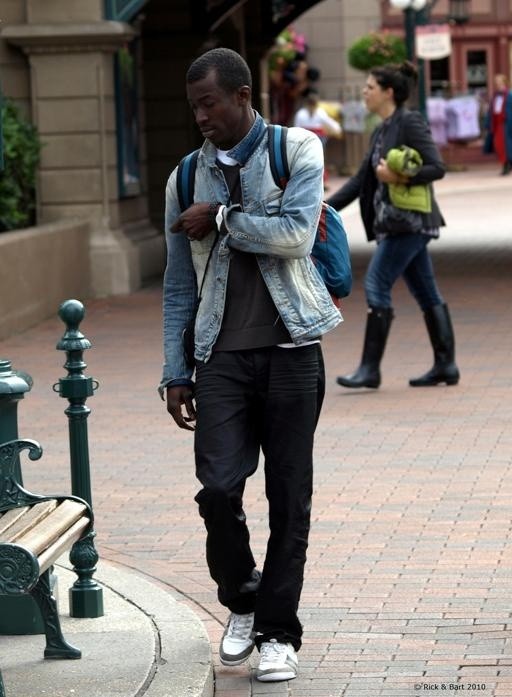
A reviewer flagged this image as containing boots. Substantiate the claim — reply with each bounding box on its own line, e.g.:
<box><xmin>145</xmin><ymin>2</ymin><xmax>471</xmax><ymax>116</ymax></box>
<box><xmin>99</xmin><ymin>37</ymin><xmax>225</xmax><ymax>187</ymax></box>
<box><xmin>410</xmin><ymin>302</ymin><xmax>460</xmax><ymax>387</ymax></box>
<box><xmin>337</xmin><ymin>307</ymin><xmax>391</xmax><ymax>388</ymax></box>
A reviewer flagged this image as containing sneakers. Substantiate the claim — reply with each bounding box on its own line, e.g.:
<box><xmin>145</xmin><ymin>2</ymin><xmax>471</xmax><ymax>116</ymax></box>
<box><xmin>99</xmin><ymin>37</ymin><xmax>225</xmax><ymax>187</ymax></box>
<box><xmin>256</xmin><ymin>640</ymin><xmax>298</xmax><ymax>681</ymax></box>
<box><xmin>219</xmin><ymin>612</ymin><xmax>256</xmax><ymax>667</ymax></box>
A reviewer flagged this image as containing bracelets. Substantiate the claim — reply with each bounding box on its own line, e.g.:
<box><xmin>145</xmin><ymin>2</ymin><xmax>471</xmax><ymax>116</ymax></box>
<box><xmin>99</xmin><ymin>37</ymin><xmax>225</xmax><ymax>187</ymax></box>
<box><xmin>208</xmin><ymin>201</ymin><xmax>222</xmax><ymax>229</ymax></box>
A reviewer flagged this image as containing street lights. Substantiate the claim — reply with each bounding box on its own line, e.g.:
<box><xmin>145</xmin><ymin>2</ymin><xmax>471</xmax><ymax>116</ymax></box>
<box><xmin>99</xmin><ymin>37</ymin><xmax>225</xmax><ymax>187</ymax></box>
<box><xmin>391</xmin><ymin>0</ymin><xmax>432</xmax><ymax>64</ymax></box>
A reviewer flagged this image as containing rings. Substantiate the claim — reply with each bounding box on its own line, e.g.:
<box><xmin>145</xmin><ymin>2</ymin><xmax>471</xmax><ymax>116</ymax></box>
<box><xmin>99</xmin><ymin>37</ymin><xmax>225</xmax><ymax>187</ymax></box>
<box><xmin>188</xmin><ymin>236</ymin><xmax>194</xmax><ymax>241</ymax></box>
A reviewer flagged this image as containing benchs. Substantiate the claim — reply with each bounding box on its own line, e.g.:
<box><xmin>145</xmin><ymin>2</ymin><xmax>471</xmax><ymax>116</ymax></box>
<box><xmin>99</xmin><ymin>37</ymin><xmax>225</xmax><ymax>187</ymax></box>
<box><xmin>0</xmin><ymin>438</ymin><xmax>94</xmax><ymax>697</ymax></box>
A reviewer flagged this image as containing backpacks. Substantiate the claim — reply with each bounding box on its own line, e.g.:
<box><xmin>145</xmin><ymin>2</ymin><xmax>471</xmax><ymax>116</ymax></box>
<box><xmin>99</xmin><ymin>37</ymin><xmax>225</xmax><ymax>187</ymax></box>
<box><xmin>174</xmin><ymin>125</ymin><xmax>351</xmax><ymax>299</ymax></box>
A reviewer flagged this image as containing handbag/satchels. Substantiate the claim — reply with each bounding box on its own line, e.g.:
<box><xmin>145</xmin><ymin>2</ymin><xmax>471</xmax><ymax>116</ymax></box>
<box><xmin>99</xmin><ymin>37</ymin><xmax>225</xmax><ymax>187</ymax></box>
<box><xmin>372</xmin><ymin>198</ymin><xmax>422</xmax><ymax>235</ymax></box>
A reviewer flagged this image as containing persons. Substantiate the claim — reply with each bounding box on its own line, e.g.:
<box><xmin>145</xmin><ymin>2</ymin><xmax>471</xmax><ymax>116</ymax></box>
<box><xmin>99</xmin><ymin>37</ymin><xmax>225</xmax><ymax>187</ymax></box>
<box><xmin>435</xmin><ymin>79</ymin><xmax>452</xmax><ymax>99</ymax></box>
<box><xmin>294</xmin><ymin>93</ymin><xmax>344</xmax><ymax>192</ymax></box>
<box><xmin>323</xmin><ymin>59</ymin><xmax>459</xmax><ymax>389</ymax></box>
<box><xmin>295</xmin><ymin>68</ymin><xmax>320</xmax><ymax>108</ymax></box>
<box><xmin>157</xmin><ymin>45</ymin><xmax>345</xmax><ymax>683</ymax></box>
<box><xmin>482</xmin><ymin>73</ymin><xmax>512</xmax><ymax>176</ymax></box>
<box><xmin>278</xmin><ymin>44</ymin><xmax>309</xmax><ymax>127</ymax></box>
